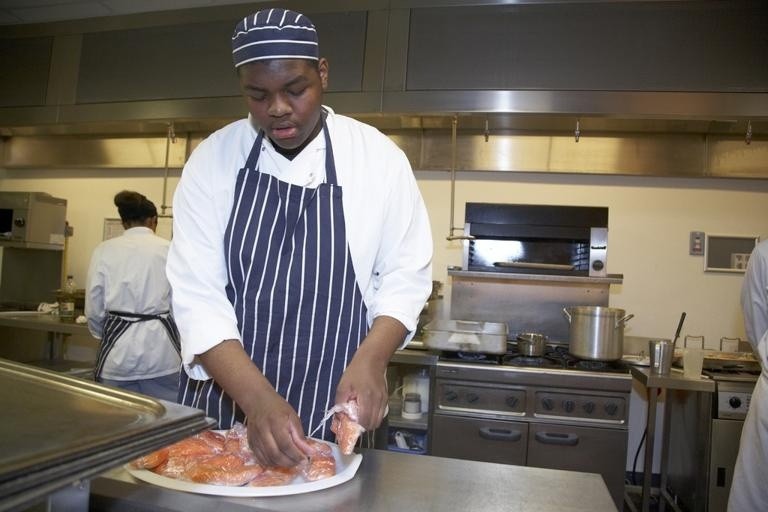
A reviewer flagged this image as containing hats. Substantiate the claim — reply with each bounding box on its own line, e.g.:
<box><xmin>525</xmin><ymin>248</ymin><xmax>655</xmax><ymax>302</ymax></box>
<box><xmin>231</xmin><ymin>8</ymin><xmax>320</xmax><ymax>68</ymax></box>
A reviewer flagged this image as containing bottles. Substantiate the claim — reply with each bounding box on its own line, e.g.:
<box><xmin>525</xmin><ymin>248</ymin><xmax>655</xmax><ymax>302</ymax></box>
<box><xmin>57</xmin><ymin>273</ymin><xmax>77</xmax><ymax>323</ymax></box>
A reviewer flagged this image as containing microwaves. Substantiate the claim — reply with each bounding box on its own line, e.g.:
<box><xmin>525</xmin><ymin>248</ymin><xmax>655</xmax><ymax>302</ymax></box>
<box><xmin>0</xmin><ymin>192</ymin><xmax>67</xmax><ymax>252</ymax></box>
<box><xmin>459</xmin><ymin>201</ymin><xmax>607</xmax><ymax>278</ymax></box>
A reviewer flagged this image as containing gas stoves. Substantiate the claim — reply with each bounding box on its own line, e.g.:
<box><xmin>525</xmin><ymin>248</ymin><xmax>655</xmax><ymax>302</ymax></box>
<box><xmin>433</xmin><ymin>349</ymin><xmax>631</xmax><ymax>415</ymax></box>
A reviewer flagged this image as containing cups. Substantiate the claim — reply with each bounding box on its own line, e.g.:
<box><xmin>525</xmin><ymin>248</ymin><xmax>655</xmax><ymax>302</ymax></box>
<box><xmin>648</xmin><ymin>339</ymin><xmax>674</xmax><ymax>375</ymax></box>
<box><xmin>402</xmin><ymin>392</ymin><xmax>420</xmax><ymax>413</ymax></box>
<box><xmin>681</xmin><ymin>348</ymin><xmax>704</xmax><ymax>379</ymax></box>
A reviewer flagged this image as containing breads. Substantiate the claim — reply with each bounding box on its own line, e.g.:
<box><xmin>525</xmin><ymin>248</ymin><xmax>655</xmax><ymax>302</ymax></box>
<box><xmin>330</xmin><ymin>411</ymin><xmax>364</xmax><ymax>455</ymax></box>
<box><xmin>131</xmin><ymin>424</ymin><xmax>335</xmax><ymax>488</ymax></box>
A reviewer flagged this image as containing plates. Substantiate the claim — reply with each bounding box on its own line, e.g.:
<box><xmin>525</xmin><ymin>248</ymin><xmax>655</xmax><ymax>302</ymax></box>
<box><xmin>492</xmin><ymin>260</ymin><xmax>574</xmax><ymax>273</ymax></box>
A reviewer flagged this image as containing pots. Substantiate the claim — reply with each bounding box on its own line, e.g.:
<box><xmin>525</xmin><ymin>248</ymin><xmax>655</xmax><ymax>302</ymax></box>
<box><xmin>420</xmin><ymin>316</ymin><xmax>509</xmax><ymax>356</ymax></box>
<box><xmin>561</xmin><ymin>301</ymin><xmax>635</xmax><ymax>364</ymax></box>
<box><xmin>513</xmin><ymin>329</ymin><xmax>543</xmax><ymax>360</ymax></box>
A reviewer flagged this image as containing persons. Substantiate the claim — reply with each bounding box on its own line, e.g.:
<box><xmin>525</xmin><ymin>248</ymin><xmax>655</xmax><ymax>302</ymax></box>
<box><xmin>84</xmin><ymin>190</ymin><xmax>182</xmax><ymax>403</ymax></box>
<box><xmin>725</xmin><ymin>239</ymin><xmax>768</xmax><ymax>512</ymax></box>
<box><xmin>165</xmin><ymin>7</ymin><xmax>433</xmax><ymax>471</ymax></box>
